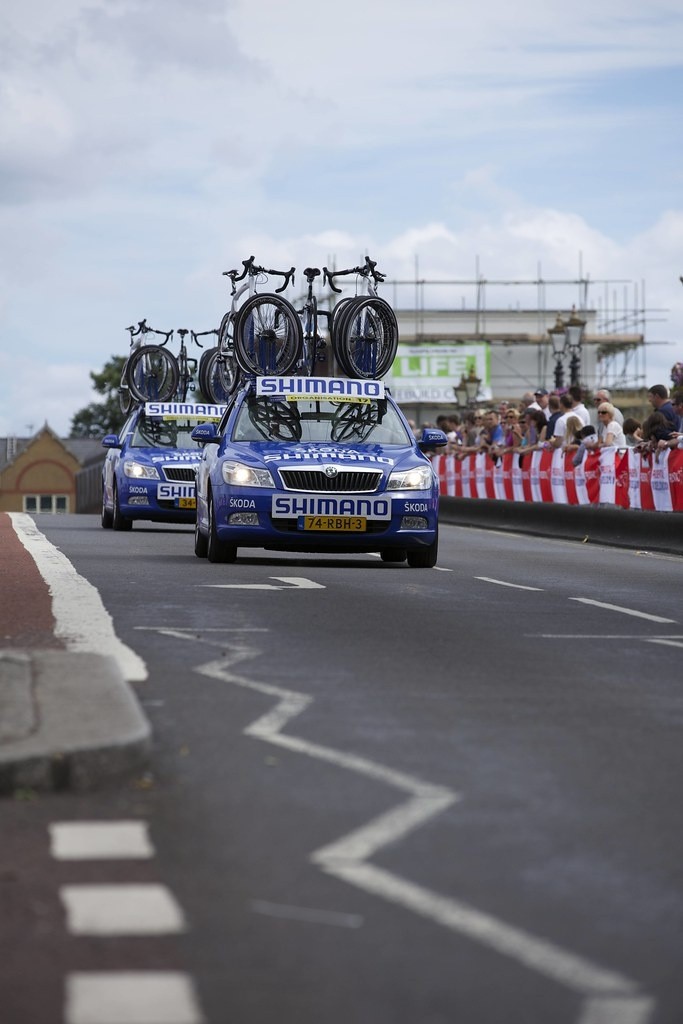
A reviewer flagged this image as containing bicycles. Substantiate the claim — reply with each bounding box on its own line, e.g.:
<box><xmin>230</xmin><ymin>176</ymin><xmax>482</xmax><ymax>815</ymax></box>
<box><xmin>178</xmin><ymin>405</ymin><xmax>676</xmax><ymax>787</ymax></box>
<box><xmin>118</xmin><ymin>255</ymin><xmax>399</xmax><ymax>418</ymax></box>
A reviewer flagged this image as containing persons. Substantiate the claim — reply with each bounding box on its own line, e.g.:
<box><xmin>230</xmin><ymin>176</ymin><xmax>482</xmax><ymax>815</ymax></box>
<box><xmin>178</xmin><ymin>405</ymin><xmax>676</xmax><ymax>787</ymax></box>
<box><xmin>406</xmin><ymin>362</ymin><xmax>683</xmax><ymax>467</ymax></box>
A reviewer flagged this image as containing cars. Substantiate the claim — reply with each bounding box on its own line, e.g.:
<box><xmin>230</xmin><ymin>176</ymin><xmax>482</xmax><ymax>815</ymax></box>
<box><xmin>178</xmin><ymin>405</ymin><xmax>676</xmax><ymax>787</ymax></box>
<box><xmin>189</xmin><ymin>380</ymin><xmax>448</xmax><ymax>568</ymax></box>
<box><xmin>101</xmin><ymin>402</ymin><xmax>229</xmax><ymax>532</ymax></box>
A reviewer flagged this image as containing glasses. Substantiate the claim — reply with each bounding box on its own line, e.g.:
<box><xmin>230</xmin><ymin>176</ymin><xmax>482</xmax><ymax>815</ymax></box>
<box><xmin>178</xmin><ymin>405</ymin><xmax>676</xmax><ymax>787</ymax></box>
<box><xmin>473</xmin><ymin>417</ymin><xmax>481</xmax><ymax>420</ymax></box>
<box><xmin>506</xmin><ymin>416</ymin><xmax>514</xmax><ymax>419</ymax></box>
<box><xmin>519</xmin><ymin>421</ymin><xmax>526</xmax><ymax>424</ymax></box>
<box><xmin>594</xmin><ymin>398</ymin><xmax>602</xmax><ymax>401</ymax></box>
<box><xmin>672</xmin><ymin>403</ymin><xmax>677</xmax><ymax>406</ymax></box>
<box><xmin>598</xmin><ymin>411</ymin><xmax>607</xmax><ymax>415</ymax></box>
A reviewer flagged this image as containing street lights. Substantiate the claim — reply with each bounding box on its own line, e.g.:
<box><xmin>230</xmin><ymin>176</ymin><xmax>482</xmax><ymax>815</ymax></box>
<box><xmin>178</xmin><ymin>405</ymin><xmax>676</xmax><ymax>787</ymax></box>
<box><xmin>548</xmin><ymin>320</ymin><xmax>566</xmax><ymax>392</ymax></box>
<box><xmin>563</xmin><ymin>303</ymin><xmax>587</xmax><ymax>404</ymax></box>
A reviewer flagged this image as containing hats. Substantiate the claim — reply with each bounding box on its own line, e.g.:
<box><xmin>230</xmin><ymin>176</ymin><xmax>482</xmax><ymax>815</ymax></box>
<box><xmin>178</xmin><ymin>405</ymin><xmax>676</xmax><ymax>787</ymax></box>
<box><xmin>534</xmin><ymin>388</ymin><xmax>547</xmax><ymax>395</ymax></box>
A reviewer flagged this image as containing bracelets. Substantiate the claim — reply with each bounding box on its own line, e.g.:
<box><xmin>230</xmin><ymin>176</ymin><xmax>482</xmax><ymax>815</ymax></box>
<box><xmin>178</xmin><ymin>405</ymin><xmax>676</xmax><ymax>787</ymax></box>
<box><xmin>664</xmin><ymin>440</ymin><xmax>668</xmax><ymax>449</ymax></box>
<box><xmin>503</xmin><ymin>448</ymin><xmax>507</xmax><ymax>453</ymax></box>
